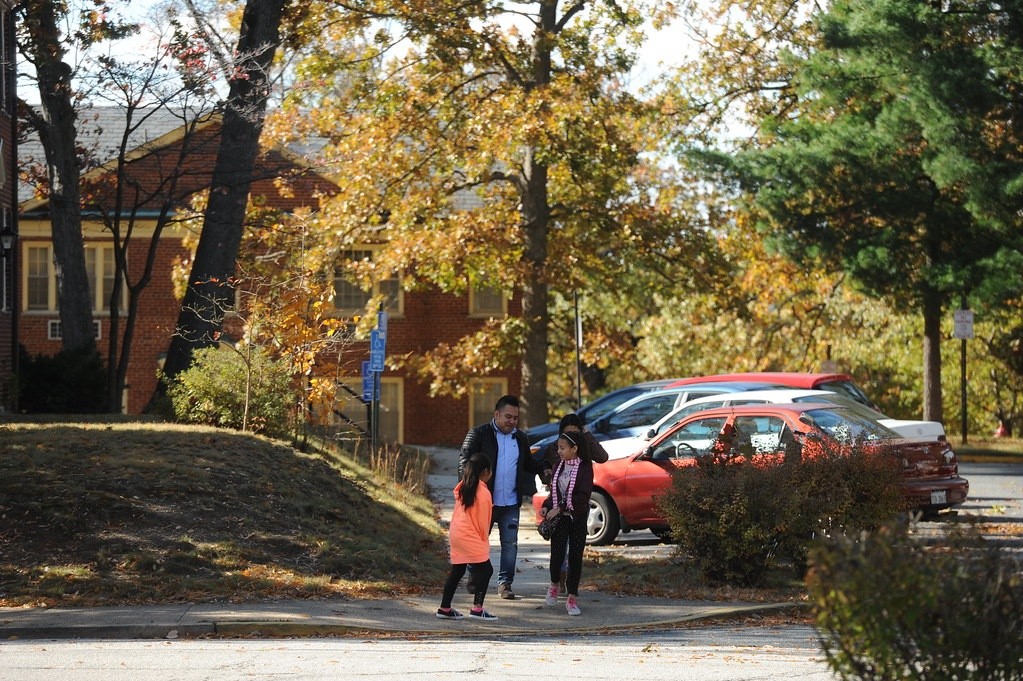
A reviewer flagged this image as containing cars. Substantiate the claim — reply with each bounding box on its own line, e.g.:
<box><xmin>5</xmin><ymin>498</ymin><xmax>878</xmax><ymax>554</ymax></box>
<box><xmin>524</xmin><ymin>372</ymin><xmax>969</xmax><ymax>546</ymax></box>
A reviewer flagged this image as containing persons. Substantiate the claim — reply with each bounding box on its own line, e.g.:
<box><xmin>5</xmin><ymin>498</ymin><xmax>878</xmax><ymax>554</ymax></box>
<box><xmin>436</xmin><ymin>453</ymin><xmax>498</xmax><ymax>621</ymax></box>
<box><xmin>538</xmin><ymin>413</ymin><xmax>609</xmax><ymax>595</ymax></box>
<box><xmin>457</xmin><ymin>395</ymin><xmax>537</xmax><ymax>598</ymax></box>
<box><xmin>538</xmin><ymin>430</ymin><xmax>595</xmax><ymax>616</ymax></box>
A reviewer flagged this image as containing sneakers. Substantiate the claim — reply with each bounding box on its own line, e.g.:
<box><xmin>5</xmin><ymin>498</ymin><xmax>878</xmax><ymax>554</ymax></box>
<box><xmin>545</xmin><ymin>586</ymin><xmax>558</xmax><ymax>606</ymax></box>
<box><xmin>469</xmin><ymin>609</ymin><xmax>498</xmax><ymax>621</ymax></box>
<box><xmin>436</xmin><ymin>608</ymin><xmax>465</xmax><ymax>621</ymax></box>
<box><xmin>565</xmin><ymin>597</ymin><xmax>581</xmax><ymax>616</ymax></box>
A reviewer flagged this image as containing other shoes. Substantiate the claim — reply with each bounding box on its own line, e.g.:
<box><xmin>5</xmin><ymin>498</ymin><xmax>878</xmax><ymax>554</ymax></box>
<box><xmin>467</xmin><ymin>573</ymin><xmax>476</xmax><ymax>594</ymax></box>
<box><xmin>498</xmin><ymin>583</ymin><xmax>514</xmax><ymax>599</ymax></box>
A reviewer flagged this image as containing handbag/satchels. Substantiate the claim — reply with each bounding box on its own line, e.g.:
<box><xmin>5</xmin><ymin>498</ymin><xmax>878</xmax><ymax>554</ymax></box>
<box><xmin>536</xmin><ymin>514</ymin><xmax>561</xmax><ymax>541</ymax></box>
